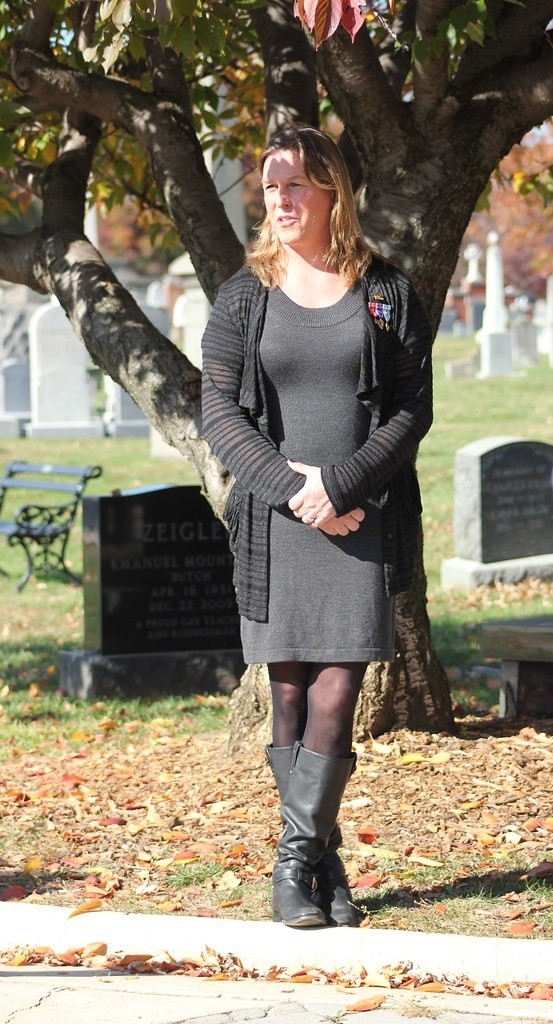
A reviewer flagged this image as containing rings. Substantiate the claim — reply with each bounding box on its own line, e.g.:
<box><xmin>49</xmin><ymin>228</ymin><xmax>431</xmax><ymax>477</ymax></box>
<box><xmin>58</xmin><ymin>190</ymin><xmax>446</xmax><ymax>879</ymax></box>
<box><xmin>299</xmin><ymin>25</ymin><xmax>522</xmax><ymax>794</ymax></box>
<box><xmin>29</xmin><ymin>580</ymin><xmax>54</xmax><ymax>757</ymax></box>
<box><xmin>306</xmin><ymin>516</ymin><xmax>314</xmax><ymax>523</ymax></box>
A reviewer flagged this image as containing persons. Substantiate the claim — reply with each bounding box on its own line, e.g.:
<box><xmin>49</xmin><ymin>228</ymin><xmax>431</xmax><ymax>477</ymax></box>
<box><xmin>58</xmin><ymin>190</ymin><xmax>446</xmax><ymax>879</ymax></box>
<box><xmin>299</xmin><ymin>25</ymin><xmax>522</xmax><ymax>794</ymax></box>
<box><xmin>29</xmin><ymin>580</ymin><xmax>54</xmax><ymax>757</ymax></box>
<box><xmin>202</xmin><ymin>126</ymin><xmax>432</xmax><ymax>927</ymax></box>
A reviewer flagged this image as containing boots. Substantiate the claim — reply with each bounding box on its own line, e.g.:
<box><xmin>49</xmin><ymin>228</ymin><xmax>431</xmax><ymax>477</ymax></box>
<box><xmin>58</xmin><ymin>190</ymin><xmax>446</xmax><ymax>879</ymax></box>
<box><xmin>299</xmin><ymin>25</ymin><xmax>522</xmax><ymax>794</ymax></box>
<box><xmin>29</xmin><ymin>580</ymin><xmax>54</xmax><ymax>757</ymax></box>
<box><xmin>265</xmin><ymin>742</ymin><xmax>356</xmax><ymax>927</ymax></box>
<box><xmin>270</xmin><ymin>741</ymin><xmax>357</xmax><ymax>927</ymax></box>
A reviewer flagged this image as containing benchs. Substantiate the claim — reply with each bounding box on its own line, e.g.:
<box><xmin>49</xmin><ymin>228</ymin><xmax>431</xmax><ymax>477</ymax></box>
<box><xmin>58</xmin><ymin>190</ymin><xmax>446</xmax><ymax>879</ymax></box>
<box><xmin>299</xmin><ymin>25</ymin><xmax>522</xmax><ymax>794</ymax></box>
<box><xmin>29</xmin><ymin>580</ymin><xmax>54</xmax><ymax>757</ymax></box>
<box><xmin>0</xmin><ymin>460</ymin><xmax>103</xmax><ymax>593</ymax></box>
